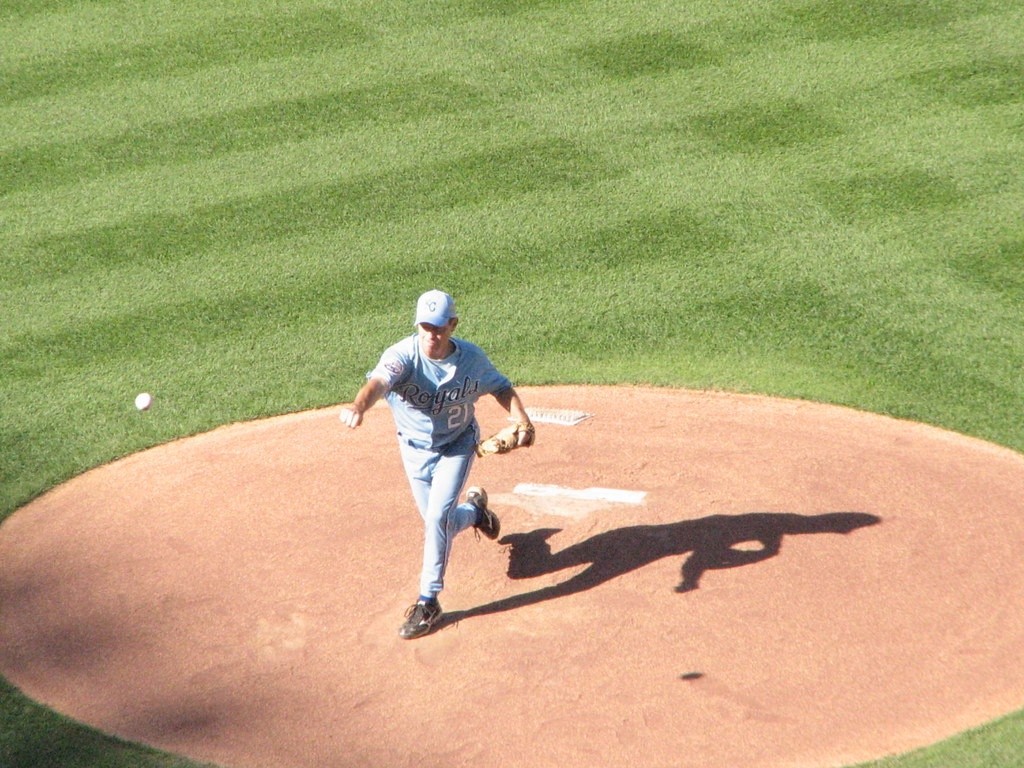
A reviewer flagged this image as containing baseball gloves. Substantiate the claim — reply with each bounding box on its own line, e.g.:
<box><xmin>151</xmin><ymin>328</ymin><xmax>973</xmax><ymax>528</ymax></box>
<box><xmin>475</xmin><ymin>421</ymin><xmax>536</xmax><ymax>458</ymax></box>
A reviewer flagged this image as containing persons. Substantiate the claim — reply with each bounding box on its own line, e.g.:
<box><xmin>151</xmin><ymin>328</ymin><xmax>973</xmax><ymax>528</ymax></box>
<box><xmin>340</xmin><ymin>289</ymin><xmax>535</xmax><ymax>639</ymax></box>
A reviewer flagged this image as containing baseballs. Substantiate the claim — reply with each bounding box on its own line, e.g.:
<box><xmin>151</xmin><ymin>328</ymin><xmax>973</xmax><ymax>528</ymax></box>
<box><xmin>135</xmin><ymin>392</ymin><xmax>152</xmax><ymax>411</ymax></box>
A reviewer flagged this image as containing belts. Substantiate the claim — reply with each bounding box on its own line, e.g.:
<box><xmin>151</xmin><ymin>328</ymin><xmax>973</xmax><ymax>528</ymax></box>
<box><xmin>398</xmin><ymin>425</ymin><xmax>473</xmax><ymax>453</ymax></box>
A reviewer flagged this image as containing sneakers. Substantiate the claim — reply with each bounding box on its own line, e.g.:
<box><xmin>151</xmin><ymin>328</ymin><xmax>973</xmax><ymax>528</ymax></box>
<box><xmin>399</xmin><ymin>597</ymin><xmax>443</xmax><ymax>638</ymax></box>
<box><xmin>466</xmin><ymin>486</ymin><xmax>500</xmax><ymax>540</ymax></box>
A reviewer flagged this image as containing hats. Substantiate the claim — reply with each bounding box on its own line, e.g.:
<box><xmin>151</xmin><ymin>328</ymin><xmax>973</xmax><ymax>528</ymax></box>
<box><xmin>414</xmin><ymin>290</ymin><xmax>455</xmax><ymax>327</ymax></box>
